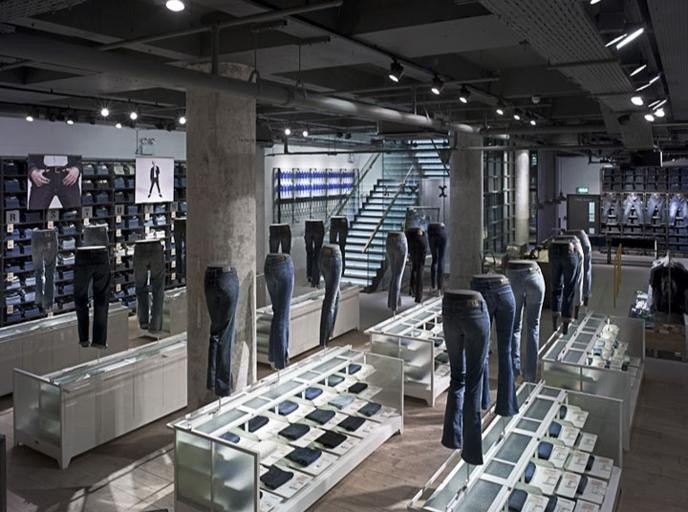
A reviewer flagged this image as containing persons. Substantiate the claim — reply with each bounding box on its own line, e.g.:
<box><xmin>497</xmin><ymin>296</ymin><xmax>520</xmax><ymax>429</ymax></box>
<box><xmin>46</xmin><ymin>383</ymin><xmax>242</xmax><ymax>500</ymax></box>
<box><xmin>28</xmin><ymin>154</ymin><xmax>83</xmax><ymax>209</ymax></box>
<box><xmin>147</xmin><ymin>161</ymin><xmax>162</xmax><ymax>198</ymax></box>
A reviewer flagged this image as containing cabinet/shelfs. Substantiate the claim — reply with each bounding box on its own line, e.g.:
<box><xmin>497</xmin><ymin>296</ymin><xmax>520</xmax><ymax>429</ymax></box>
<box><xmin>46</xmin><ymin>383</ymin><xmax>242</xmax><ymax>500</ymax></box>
<box><xmin>1</xmin><ymin>156</ymin><xmax>186</xmax><ymax>328</ymax></box>
<box><xmin>406</xmin><ymin>378</ymin><xmax>624</xmax><ymax>511</ymax></box>
<box><xmin>363</xmin><ymin>288</ymin><xmax>451</xmax><ymax>408</ymax></box>
<box><xmin>256</xmin><ymin>284</ymin><xmax>361</xmax><ymax>365</ymax></box>
<box><xmin>538</xmin><ymin>305</ymin><xmax>645</xmax><ymax>453</ymax></box>
<box><xmin>166</xmin><ymin>343</ymin><xmax>404</xmax><ymax>512</ymax></box>
<box><xmin>12</xmin><ymin>331</ymin><xmax>188</xmax><ymax>470</ymax></box>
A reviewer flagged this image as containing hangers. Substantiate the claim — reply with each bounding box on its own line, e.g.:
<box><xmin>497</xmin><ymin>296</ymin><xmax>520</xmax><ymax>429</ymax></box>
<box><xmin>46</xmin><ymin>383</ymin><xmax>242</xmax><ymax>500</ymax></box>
<box><xmin>649</xmin><ymin>249</ymin><xmax>688</xmax><ymax>275</ymax></box>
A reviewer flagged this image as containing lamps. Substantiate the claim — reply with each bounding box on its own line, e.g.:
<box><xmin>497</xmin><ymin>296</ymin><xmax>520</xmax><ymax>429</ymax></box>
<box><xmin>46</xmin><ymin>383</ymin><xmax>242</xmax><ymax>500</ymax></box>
<box><xmin>530</xmin><ymin>150</ymin><xmax>567</xmax><ymax>209</ymax></box>
